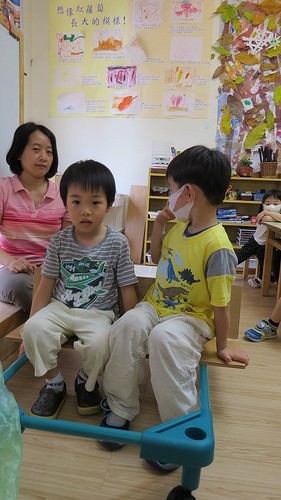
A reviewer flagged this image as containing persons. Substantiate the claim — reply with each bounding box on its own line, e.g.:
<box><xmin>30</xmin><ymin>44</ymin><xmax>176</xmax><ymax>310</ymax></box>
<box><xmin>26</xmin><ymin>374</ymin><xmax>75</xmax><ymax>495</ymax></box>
<box><xmin>1</xmin><ymin>122</ymin><xmax>72</xmax><ymax>317</ymax></box>
<box><xmin>19</xmin><ymin>160</ymin><xmax>139</xmax><ymax>421</ymax></box>
<box><xmin>236</xmin><ymin>189</ymin><xmax>281</xmax><ymax>288</ymax></box>
<box><xmin>100</xmin><ymin>145</ymin><xmax>250</xmax><ymax>472</ymax></box>
<box><xmin>244</xmin><ymin>297</ymin><xmax>281</xmax><ymax>342</ymax></box>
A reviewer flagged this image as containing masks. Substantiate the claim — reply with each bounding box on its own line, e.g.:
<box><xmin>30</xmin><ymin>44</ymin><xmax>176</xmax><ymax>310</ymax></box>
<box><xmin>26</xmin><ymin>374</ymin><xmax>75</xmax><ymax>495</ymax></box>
<box><xmin>168</xmin><ymin>191</ymin><xmax>192</xmax><ymax>222</ymax></box>
<box><xmin>262</xmin><ymin>204</ymin><xmax>281</xmax><ymax>214</ymax></box>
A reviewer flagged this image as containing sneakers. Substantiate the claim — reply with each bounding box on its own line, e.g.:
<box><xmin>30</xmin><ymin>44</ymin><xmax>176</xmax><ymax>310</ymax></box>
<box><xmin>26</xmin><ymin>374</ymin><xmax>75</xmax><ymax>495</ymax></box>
<box><xmin>244</xmin><ymin>319</ymin><xmax>277</xmax><ymax>342</ymax></box>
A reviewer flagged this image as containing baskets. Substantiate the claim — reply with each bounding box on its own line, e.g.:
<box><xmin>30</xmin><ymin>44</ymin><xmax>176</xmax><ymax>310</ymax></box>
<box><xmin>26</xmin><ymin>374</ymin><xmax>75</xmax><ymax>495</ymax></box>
<box><xmin>260</xmin><ymin>162</ymin><xmax>278</xmax><ymax>178</ymax></box>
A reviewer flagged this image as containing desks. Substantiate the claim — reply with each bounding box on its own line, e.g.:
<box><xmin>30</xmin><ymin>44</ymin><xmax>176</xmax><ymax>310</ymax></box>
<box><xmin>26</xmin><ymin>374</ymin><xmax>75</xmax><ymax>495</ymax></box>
<box><xmin>259</xmin><ymin>221</ymin><xmax>281</xmax><ymax>303</ymax></box>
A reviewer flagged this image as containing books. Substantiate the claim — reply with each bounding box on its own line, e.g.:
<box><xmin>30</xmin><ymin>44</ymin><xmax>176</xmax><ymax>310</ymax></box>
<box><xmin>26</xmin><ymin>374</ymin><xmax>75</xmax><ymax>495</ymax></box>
<box><xmin>216</xmin><ymin>207</ymin><xmax>257</xmax><ymax>224</ymax></box>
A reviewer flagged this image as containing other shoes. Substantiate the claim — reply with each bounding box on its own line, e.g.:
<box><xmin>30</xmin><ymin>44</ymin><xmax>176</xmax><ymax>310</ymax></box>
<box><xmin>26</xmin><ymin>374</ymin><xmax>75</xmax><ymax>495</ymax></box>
<box><xmin>145</xmin><ymin>459</ymin><xmax>181</xmax><ymax>472</ymax></box>
<box><xmin>247</xmin><ymin>277</ymin><xmax>262</xmax><ymax>288</ymax></box>
<box><xmin>95</xmin><ymin>397</ymin><xmax>130</xmax><ymax>452</ymax></box>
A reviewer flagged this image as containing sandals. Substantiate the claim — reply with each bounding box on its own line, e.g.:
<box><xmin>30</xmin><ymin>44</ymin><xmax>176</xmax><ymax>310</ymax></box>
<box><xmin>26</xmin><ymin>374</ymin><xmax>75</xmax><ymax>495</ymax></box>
<box><xmin>74</xmin><ymin>376</ymin><xmax>102</xmax><ymax>416</ymax></box>
<box><xmin>27</xmin><ymin>380</ymin><xmax>68</xmax><ymax>420</ymax></box>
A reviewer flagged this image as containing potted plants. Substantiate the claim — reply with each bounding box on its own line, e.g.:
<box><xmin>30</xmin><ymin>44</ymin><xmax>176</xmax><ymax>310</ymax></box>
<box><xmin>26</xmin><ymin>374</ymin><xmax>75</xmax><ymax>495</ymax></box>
<box><xmin>238</xmin><ymin>152</ymin><xmax>253</xmax><ymax>176</ymax></box>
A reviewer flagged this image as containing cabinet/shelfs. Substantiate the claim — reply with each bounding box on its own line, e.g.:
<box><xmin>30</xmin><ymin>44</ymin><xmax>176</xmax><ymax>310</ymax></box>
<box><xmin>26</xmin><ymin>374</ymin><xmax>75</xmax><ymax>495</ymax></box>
<box><xmin>143</xmin><ymin>167</ymin><xmax>281</xmax><ymax>272</ymax></box>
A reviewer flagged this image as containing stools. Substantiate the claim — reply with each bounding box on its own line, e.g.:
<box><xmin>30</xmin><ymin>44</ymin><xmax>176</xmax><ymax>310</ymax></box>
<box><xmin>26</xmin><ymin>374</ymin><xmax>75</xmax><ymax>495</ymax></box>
<box><xmin>243</xmin><ymin>255</ymin><xmax>258</xmax><ymax>281</ymax></box>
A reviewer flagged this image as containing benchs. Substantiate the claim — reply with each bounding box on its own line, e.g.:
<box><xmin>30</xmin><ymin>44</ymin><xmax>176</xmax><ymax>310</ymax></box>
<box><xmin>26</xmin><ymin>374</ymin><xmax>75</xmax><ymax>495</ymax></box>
<box><xmin>4</xmin><ymin>267</ymin><xmax>247</xmax><ymax>370</ymax></box>
